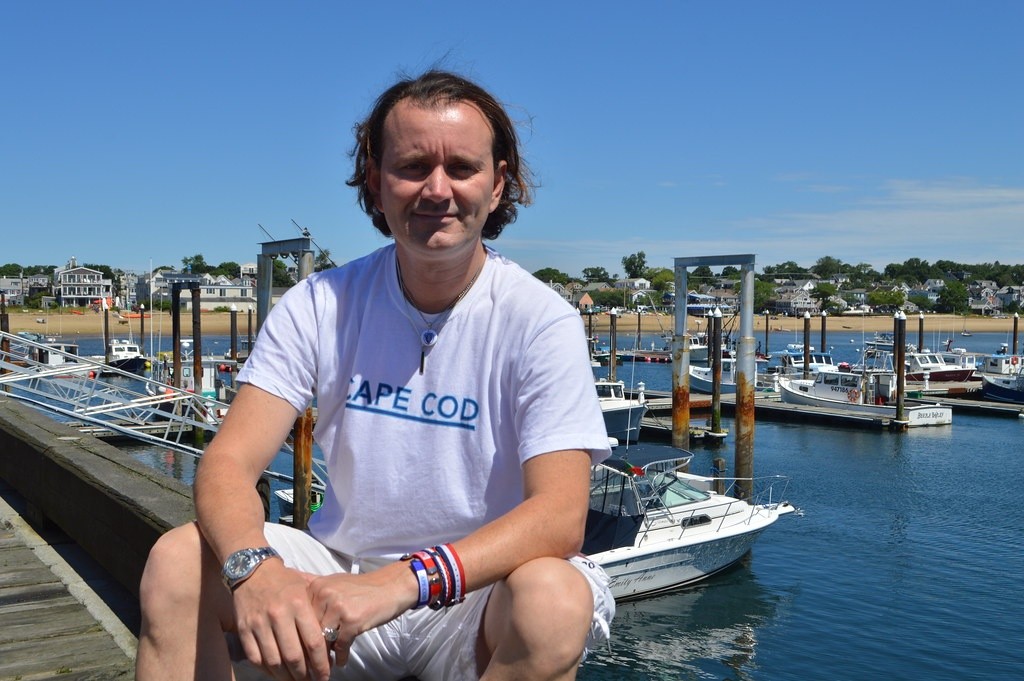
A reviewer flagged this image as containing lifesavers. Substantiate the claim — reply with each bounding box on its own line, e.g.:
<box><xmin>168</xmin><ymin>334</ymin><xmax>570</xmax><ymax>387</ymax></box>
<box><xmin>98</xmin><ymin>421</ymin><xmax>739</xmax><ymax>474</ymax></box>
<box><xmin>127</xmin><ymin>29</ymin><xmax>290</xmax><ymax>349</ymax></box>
<box><xmin>847</xmin><ymin>389</ymin><xmax>860</xmax><ymax>403</ymax></box>
<box><xmin>1010</xmin><ymin>355</ymin><xmax>1017</xmax><ymax>365</ymax></box>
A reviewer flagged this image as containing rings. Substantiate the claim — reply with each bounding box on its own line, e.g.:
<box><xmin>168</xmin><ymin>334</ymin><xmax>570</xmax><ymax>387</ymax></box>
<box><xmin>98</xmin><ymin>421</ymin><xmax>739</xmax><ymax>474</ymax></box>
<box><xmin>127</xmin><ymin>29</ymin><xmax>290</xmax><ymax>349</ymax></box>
<box><xmin>321</xmin><ymin>625</ymin><xmax>339</xmax><ymax>641</ymax></box>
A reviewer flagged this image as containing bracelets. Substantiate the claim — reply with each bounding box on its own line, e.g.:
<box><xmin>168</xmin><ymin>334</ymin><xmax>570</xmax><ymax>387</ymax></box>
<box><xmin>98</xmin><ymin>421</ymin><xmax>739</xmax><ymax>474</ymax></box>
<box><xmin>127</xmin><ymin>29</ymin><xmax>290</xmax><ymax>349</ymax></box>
<box><xmin>401</xmin><ymin>543</ymin><xmax>466</xmax><ymax>610</ymax></box>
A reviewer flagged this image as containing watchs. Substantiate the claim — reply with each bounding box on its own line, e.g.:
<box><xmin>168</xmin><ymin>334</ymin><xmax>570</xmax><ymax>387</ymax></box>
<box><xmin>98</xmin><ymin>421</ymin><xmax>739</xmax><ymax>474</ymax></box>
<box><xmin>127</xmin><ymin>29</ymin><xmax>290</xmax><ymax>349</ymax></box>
<box><xmin>220</xmin><ymin>547</ymin><xmax>284</xmax><ymax>595</ymax></box>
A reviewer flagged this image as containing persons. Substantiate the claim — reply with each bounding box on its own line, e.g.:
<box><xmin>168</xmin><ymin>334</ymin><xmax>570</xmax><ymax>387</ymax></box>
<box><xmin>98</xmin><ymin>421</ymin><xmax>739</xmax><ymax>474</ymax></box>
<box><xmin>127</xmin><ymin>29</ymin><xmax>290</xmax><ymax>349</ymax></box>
<box><xmin>136</xmin><ymin>71</ymin><xmax>612</xmax><ymax>681</ymax></box>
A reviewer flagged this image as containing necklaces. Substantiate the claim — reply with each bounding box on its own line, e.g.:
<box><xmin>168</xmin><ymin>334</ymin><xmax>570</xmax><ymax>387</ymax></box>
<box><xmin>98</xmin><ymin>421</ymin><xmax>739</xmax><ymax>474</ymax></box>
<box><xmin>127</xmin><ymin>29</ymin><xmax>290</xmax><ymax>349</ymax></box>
<box><xmin>395</xmin><ymin>256</ymin><xmax>484</xmax><ymax>374</ymax></box>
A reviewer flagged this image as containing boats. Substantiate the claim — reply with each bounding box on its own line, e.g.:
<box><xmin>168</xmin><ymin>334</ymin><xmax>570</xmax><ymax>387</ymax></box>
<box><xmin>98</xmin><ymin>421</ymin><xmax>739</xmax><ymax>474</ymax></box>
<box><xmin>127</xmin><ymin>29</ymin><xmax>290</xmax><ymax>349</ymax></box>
<box><xmin>593</xmin><ymin>377</ymin><xmax>650</xmax><ymax>446</ymax></box>
<box><xmin>581</xmin><ymin>441</ymin><xmax>797</xmax><ymax>604</ymax></box>
<box><xmin>579</xmin><ymin>305</ymin><xmax>1023</xmax><ymax>429</ymax></box>
<box><xmin>17</xmin><ymin>303</ymin><xmax>256</xmax><ymax>426</ymax></box>
